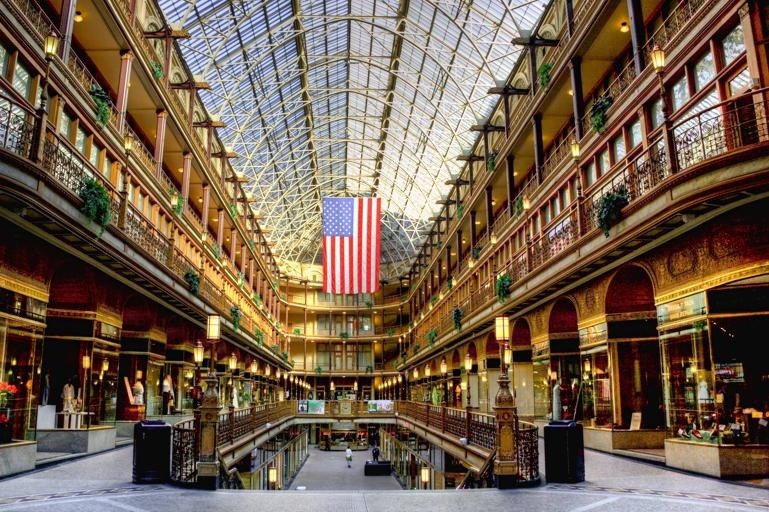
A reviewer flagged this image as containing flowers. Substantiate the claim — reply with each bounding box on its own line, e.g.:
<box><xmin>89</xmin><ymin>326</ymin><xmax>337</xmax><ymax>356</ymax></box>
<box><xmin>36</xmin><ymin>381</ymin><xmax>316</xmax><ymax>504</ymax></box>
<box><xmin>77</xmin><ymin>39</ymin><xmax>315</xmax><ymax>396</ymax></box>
<box><xmin>0</xmin><ymin>378</ymin><xmax>17</xmax><ymax>408</ymax></box>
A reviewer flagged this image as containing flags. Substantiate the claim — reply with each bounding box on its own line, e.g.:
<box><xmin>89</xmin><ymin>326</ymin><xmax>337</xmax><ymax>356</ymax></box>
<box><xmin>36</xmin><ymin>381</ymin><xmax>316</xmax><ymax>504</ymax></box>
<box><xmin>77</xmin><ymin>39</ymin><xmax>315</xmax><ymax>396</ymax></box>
<box><xmin>320</xmin><ymin>195</ymin><xmax>383</xmax><ymax>296</ymax></box>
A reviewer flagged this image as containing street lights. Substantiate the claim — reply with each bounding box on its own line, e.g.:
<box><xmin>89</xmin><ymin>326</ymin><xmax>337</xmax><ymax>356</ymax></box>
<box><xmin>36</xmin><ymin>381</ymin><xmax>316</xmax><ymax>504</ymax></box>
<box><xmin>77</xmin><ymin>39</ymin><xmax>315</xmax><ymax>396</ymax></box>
<box><xmin>250</xmin><ymin>358</ymin><xmax>258</xmax><ymax>434</ymax></box>
<box><xmin>194</xmin><ymin>338</ymin><xmax>205</xmax><ymax>441</ymax></box>
<box><xmin>490</xmin><ymin>231</ymin><xmax>499</xmax><ymax>299</ymax></box>
<box><xmin>222</xmin><ymin>252</ymin><xmax>229</xmax><ymax>313</ymax></box>
<box><xmin>196</xmin><ymin>312</ymin><xmax>222</xmax><ymax>490</ymax></box>
<box><xmin>467</xmin><ymin>258</ymin><xmax>475</xmax><ymax>314</ymax></box>
<box><xmin>523</xmin><ymin>193</ymin><xmax>534</xmax><ymax>273</ymax></box>
<box><xmin>263</xmin><ymin>363</ymin><xmax>272</xmax><ymax>423</ymax></box>
<box><xmin>451</xmin><ymin>276</ymin><xmax>458</xmax><ymax>324</ymax></box>
<box><xmin>570</xmin><ymin>138</ymin><xmax>587</xmax><ymax>239</ymax></box>
<box><xmin>439</xmin><ymin>358</ymin><xmax>448</xmax><ymax>429</ymax></box>
<box><xmin>585</xmin><ymin>357</ymin><xmax>591</xmax><ymax>404</ymax></box>
<box><xmin>237</xmin><ymin>275</ymin><xmax>287</xmax><ymax>354</ymax></box>
<box><xmin>463</xmin><ymin>352</ymin><xmax>475</xmax><ymax>444</ymax></box>
<box><xmin>100</xmin><ymin>357</ymin><xmax>109</xmax><ymax>421</ymax></box>
<box><xmin>500</xmin><ymin>343</ymin><xmax>512</xmax><ymax>386</ymax></box>
<box><xmin>398</xmin><ymin>288</ymin><xmax>444</xmax><ymax>361</ymax></box>
<box><xmin>228</xmin><ymin>352</ymin><xmax>238</xmax><ymax>440</ymax></box>
<box><xmin>269</xmin><ymin>467</ymin><xmax>277</xmax><ymax>490</ymax></box>
<box><xmin>651</xmin><ymin>38</ymin><xmax>682</xmax><ymax>180</ymax></box>
<box><xmin>117</xmin><ymin>129</ymin><xmax>134</xmax><ymax>232</ymax></box>
<box><xmin>199</xmin><ymin>229</ymin><xmax>209</xmax><ymax>294</ymax></box>
<box><xmin>166</xmin><ymin>190</ymin><xmax>181</xmax><ymax>270</ymax></box>
<box><xmin>424</xmin><ymin>364</ymin><xmax>431</xmax><ymax>426</ymax></box>
<box><xmin>493</xmin><ymin>312</ymin><xmax>518</xmax><ymax>489</ymax></box>
<box><xmin>276</xmin><ymin>366</ymin><xmax>419</xmax><ymax>423</ymax></box>
<box><xmin>30</xmin><ymin>29</ymin><xmax>61</xmax><ymax>166</ymax></box>
<box><xmin>420</xmin><ymin>462</ymin><xmax>430</xmax><ymax>490</ymax></box>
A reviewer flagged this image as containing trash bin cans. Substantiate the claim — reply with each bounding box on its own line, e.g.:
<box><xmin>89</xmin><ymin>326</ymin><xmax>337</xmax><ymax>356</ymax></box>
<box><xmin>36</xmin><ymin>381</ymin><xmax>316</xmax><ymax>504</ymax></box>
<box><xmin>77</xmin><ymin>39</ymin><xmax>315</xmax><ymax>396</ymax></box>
<box><xmin>132</xmin><ymin>420</ymin><xmax>171</xmax><ymax>484</ymax></box>
<box><xmin>544</xmin><ymin>420</ymin><xmax>585</xmax><ymax>483</ymax></box>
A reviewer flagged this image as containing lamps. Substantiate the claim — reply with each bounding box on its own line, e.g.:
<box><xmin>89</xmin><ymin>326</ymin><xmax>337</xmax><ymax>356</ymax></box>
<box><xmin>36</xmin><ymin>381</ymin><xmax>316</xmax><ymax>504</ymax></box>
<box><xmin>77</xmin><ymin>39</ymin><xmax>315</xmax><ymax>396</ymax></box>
<box><xmin>420</xmin><ymin>463</ymin><xmax>431</xmax><ymax>488</ymax></box>
<box><xmin>168</xmin><ymin>187</ymin><xmax>181</xmax><ymax>210</ymax></box>
<box><xmin>198</xmin><ymin>227</ymin><xmax>244</xmax><ymax>291</ymax></box>
<box><xmin>189</xmin><ymin>311</ymin><xmax>305</xmax><ymax>403</ymax></box>
<box><xmin>649</xmin><ymin>41</ymin><xmax>669</xmax><ymax>98</ymax></box>
<box><xmin>122</xmin><ymin>132</ymin><xmax>135</xmax><ymax>161</ymax></box>
<box><xmin>376</xmin><ymin>310</ymin><xmax>519</xmax><ymax>402</ymax></box>
<box><xmin>73</xmin><ymin>9</ymin><xmax>84</xmax><ymax>24</ymax></box>
<box><xmin>570</xmin><ymin>136</ymin><xmax>582</xmax><ymax>162</ymax></box>
<box><xmin>36</xmin><ymin>26</ymin><xmax>63</xmax><ymax>110</ymax></box>
<box><xmin>457</xmin><ymin>193</ymin><xmax>534</xmax><ymax>272</ymax></box>
<box><xmin>268</xmin><ymin>465</ymin><xmax>279</xmax><ymax>489</ymax></box>
<box><xmin>620</xmin><ymin>20</ymin><xmax>629</xmax><ymax>34</ymax></box>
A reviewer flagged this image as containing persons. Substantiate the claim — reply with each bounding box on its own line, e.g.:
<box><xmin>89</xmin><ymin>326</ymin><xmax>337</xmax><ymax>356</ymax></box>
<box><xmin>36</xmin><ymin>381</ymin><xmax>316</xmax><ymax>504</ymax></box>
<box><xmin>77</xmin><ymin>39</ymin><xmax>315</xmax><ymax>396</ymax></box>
<box><xmin>344</xmin><ymin>445</ymin><xmax>354</xmax><ymax>468</ymax></box>
<box><xmin>61</xmin><ymin>377</ymin><xmax>75</xmax><ymax>412</ymax></box>
<box><xmin>371</xmin><ymin>445</ymin><xmax>380</xmax><ymax>461</ymax></box>
<box><xmin>551</xmin><ymin>383</ymin><xmax>565</xmax><ymax>421</ymax></box>
<box><xmin>134</xmin><ymin>380</ymin><xmax>145</xmax><ymax>405</ymax></box>
<box><xmin>161</xmin><ymin>374</ymin><xmax>174</xmax><ymax>415</ymax></box>
